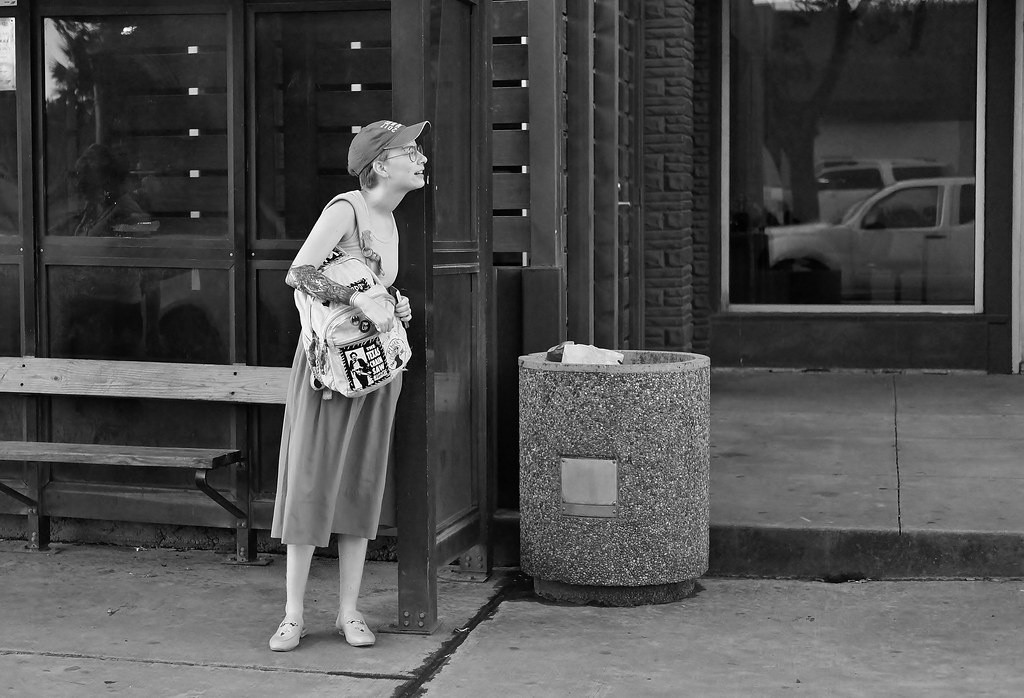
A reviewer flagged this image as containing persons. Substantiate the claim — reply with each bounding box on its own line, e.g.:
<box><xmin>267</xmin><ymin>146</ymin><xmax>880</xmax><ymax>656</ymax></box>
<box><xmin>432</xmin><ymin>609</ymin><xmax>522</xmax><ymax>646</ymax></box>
<box><xmin>66</xmin><ymin>141</ymin><xmax>144</xmax><ymax>484</ymax></box>
<box><xmin>264</xmin><ymin>121</ymin><xmax>432</xmax><ymax>652</ymax></box>
<box><xmin>350</xmin><ymin>352</ymin><xmax>369</xmax><ymax>388</ymax></box>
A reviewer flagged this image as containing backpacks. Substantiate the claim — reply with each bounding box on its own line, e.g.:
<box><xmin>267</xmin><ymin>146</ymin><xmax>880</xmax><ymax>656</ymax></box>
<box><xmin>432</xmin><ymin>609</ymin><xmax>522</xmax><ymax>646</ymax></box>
<box><xmin>293</xmin><ymin>195</ymin><xmax>413</xmax><ymax>400</ymax></box>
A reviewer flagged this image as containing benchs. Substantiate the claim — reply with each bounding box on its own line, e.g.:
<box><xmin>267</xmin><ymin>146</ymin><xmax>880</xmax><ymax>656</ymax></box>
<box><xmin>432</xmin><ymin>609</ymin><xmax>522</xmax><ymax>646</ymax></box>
<box><xmin>0</xmin><ymin>356</ymin><xmax>293</xmax><ymax>568</ymax></box>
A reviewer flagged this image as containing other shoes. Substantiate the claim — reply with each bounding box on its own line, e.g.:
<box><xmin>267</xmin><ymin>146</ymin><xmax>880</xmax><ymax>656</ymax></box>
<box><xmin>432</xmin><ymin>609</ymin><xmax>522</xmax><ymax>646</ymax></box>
<box><xmin>269</xmin><ymin>618</ymin><xmax>308</xmax><ymax>652</ymax></box>
<box><xmin>335</xmin><ymin>609</ymin><xmax>376</xmax><ymax>647</ymax></box>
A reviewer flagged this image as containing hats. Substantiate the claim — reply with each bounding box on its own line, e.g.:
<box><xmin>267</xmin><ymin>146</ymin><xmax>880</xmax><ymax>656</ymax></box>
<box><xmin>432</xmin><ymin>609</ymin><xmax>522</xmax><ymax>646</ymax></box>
<box><xmin>347</xmin><ymin>120</ymin><xmax>432</xmax><ymax>180</ymax></box>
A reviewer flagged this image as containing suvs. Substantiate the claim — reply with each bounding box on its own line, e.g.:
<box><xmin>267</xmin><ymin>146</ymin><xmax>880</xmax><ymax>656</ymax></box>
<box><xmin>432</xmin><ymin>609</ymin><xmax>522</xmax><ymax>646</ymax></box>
<box><xmin>764</xmin><ymin>157</ymin><xmax>958</xmax><ymax>225</ymax></box>
<box><xmin>749</xmin><ymin>172</ymin><xmax>976</xmax><ymax>302</ymax></box>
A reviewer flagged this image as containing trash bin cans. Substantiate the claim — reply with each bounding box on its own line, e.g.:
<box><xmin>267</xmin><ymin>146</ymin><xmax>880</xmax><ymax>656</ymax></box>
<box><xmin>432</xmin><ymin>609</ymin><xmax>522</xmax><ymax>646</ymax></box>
<box><xmin>520</xmin><ymin>350</ymin><xmax>712</xmax><ymax>605</ymax></box>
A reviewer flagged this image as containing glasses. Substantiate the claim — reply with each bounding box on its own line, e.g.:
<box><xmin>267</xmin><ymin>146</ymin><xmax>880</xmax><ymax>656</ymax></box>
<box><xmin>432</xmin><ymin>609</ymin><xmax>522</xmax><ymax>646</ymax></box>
<box><xmin>384</xmin><ymin>144</ymin><xmax>424</xmax><ymax>163</ymax></box>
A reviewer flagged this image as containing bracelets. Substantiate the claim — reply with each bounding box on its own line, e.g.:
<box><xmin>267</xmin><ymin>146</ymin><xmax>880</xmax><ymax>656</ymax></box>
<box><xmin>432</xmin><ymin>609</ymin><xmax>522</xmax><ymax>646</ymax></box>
<box><xmin>350</xmin><ymin>291</ymin><xmax>360</xmax><ymax>309</ymax></box>
